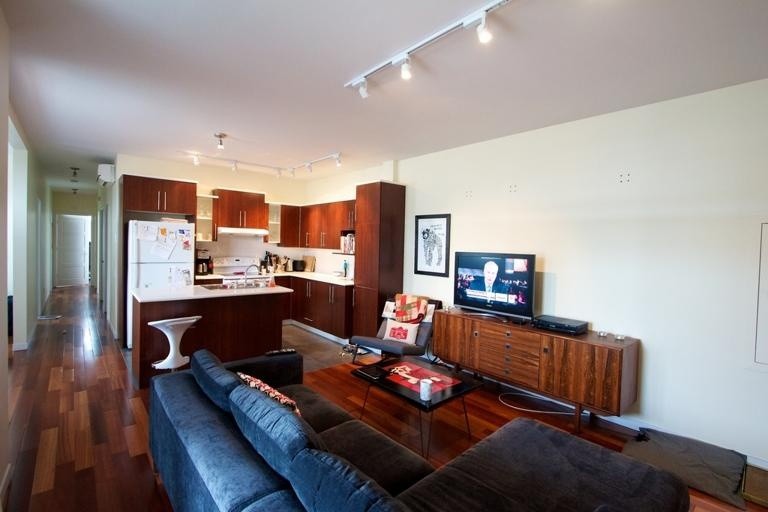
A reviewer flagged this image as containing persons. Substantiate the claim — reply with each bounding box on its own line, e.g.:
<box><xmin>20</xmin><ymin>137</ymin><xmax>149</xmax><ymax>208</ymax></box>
<box><xmin>468</xmin><ymin>260</ymin><xmax>511</xmax><ymax>294</ymax></box>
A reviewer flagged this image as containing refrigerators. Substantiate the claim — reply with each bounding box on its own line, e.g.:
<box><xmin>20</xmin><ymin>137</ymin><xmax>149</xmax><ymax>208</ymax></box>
<box><xmin>127</xmin><ymin>219</ymin><xmax>196</xmax><ymax>348</ymax></box>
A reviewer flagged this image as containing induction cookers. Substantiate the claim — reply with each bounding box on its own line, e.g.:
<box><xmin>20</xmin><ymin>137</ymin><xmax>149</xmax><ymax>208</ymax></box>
<box><xmin>217</xmin><ymin>271</ymin><xmax>269</xmax><ymax>279</ymax></box>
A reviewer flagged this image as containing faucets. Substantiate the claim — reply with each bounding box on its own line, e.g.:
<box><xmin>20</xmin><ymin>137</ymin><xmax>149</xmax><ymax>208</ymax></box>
<box><xmin>244</xmin><ymin>264</ymin><xmax>261</xmax><ymax>288</ymax></box>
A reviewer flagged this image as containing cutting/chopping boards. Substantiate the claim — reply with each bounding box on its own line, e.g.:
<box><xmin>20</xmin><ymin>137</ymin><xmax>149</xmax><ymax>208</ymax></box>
<box><xmin>303</xmin><ymin>255</ymin><xmax>315</xmax><ymax>272</ymax></box>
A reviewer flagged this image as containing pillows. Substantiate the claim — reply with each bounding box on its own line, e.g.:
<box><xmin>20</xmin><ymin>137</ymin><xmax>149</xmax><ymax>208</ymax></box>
<box><xmin>228</xmin><ymin>367</ymin><xmax>306</xmax><ymax>421</ymax></box>
<box><xmin>383</xmin><ymin>316</ymin><xmax>421</xmax><ymax>347</ymax></box>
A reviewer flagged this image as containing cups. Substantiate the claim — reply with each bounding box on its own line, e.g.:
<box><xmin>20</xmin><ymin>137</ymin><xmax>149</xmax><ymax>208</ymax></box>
<box><xmin>340</xmin><ymin>234</ymin><xmax>355</xmax><ymax>254</ymax></box>
<box><xmin>420</xmin><ymin>378</ymin><xmax>432</xmax><ymax>401</ymax></box>
<box><xmin>293</xmin><ymin>260</ymin><xmax>305</xmax><ymax>271</ymax></box>
<box><xmin>197</xmin><ymin>233</ymin><xmax>203</xmax><ymax>239</ymax></box>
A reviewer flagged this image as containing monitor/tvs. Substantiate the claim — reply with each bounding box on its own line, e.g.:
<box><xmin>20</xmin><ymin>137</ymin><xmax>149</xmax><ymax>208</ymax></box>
<box><xmin>454</xmin><ymin>252</ymin><xmax>536</xmax><ymax>322</ymax></box>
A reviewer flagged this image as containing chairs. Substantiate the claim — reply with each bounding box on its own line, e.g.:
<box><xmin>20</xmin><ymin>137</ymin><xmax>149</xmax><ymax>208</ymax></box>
<box><xmin>346</xmin><ymin>293</ymin><xmax>440</xmax><ymax>369</ymax></box>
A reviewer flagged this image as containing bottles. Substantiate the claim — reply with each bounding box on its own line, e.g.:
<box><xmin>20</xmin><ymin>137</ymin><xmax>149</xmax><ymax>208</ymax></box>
<box><xmin>260</xmin><ymin>251</ymin><xmax>294</xmax><ymax>274</ymax></box>
<box><xmin>207</xmin><ymin>231</ymin><xmax>211</xmax><ymax>240</ymax></box>
<box><xmin>347</xmin><ymin>256</ymin><xmax>352</xmax><ymax>279</ymax></box>
<box><xmin>343</xmin><ymin>259</ymin><xmax>347</xmax><ymax>277</ymax></box>
<box><xmin>269</xmin><ymin>270</ymin><xmax>275</xmax><ymax>287</ymax></box>
<box><xmin>199</xmin><ymin>205</ymin><xmax>204</xmax><ymax>216</ymax></box>
<box><xmin>205</xmin><ymin>207</ymin><xmax>209</xmax><ymax>217</ymax></box>
<box><xmin>208</xmin><ymin>256</ymin><xmax>212</xmax><ymax>274</ymax></box>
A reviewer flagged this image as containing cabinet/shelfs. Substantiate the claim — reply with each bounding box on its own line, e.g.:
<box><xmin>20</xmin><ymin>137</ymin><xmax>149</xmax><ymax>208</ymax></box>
<box><xmin>300</xmin><ymin>204</ymin><xmax>318</xmax><ymax>248</ymax></box>
<box><xmin>264</xmin><ymin>201</ymin><xmax>281</xmax><ymax>244</ymax></box>
<box><xmin>318</xmin><ymin>201</ymin><xmax>341</xmax><ymax>249</ymax></box>
<box><xmin>118</xmin><ymin>173</ymin><xmax>197</xmax><ymax>218</ymax></box>
<box><xmin>342</xmin><ymin>200</ymin><xmax>356</xmax><ymax>231</ymax></box>
<box><xmin>211</xmin><ymin>189</ymin><xmax>269</xmax><ymax>236</ymax></box>
<box><xmin>431</xmin><ymin>306</ymin><xmax>641</xmax><ymax>436</ymax></box>
<box><xmin>196</xmin><ymin>193</ymin><xmax>220</xmax><ymax>243</ymax></box>
<box><xmin>277</xmin><ymin>205</ymin><xmax>299</xmax><ymax>248</ymax></box>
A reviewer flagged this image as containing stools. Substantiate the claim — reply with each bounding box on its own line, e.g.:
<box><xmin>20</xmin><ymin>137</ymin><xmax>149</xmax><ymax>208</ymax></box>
<box><xmin>145</xmin><ymin>311</ymin><xmax>201</xmax><ymax>373</ymax></box>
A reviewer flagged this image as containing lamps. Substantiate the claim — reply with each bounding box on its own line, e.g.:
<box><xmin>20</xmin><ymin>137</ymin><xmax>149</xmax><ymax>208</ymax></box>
<box><xmin>342</xmin><ymin>0</ymin><xmax>510</xmax><ymax>104</ymax></box>
<box><xmin>182</xmin><ymin>152</ymin><xmax>345</xmax><ymax>180</ymax></box>
<box><xmin>215</xmin><ymin>133</ymin><xmax>228</xmax><ymax>151</ymax></box>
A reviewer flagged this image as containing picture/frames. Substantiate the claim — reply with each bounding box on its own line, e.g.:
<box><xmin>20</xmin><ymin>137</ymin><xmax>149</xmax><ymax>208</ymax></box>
<box><xmin>414</xmin><ymin>213</ymin><xmax>451</xmax><ymax>279</ymax></box>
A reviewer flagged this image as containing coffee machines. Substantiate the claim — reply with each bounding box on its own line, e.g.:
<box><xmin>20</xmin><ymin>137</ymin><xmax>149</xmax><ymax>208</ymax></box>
<box><xmin>195</xmin><ymin>249</ymin><xmax>210</xmax><ymax>274</ymax></box>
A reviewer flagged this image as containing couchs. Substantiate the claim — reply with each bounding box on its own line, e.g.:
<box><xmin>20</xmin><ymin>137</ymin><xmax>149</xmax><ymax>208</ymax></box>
<box><xmin>147</xmin><ymin>347</ymin><xmax>693</xmax><ymax>511</ymax></box>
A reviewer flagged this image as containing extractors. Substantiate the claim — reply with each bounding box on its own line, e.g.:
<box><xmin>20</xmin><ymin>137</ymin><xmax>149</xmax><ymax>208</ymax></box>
<box><xmin>217</xmin><ymin>226</ymin><xmax>269</xmax><ymax>237</ymax></box>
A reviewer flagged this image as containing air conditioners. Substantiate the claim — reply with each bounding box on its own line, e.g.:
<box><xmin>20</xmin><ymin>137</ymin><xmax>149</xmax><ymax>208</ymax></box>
<box><xmin>94</xmin><ymin>163</ymin><xmax>115</xmax><ymax>189</ymax></box>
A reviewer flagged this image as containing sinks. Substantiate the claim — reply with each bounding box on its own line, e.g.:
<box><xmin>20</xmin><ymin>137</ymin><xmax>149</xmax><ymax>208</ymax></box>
<box><xmin>202</xmin><ymin>284</ymin><xmax>244</xmax><ymax>291</ymax></box>
<box><xmin>238</xmin><ymin>282</ymin><xmax>271</xmax><ymax>288</ymax></box>
